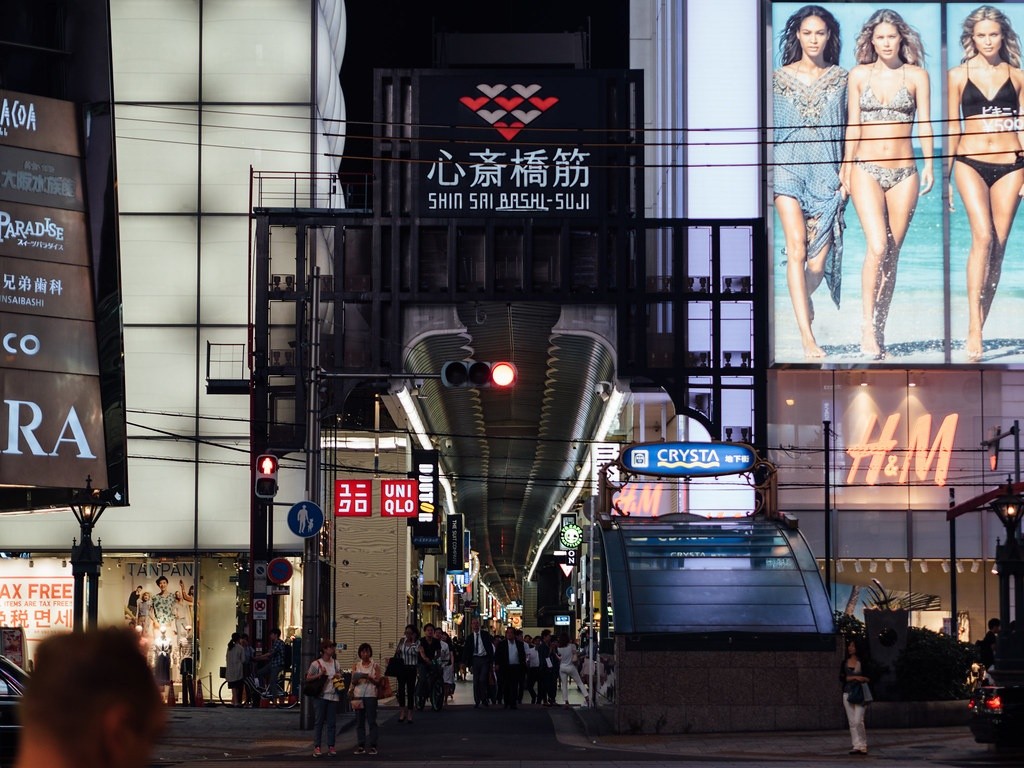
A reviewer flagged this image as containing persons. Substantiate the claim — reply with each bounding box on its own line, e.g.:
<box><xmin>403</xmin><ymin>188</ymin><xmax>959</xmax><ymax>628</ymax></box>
<box><xmin>307</xmin><ymin>641</ymin><xmax>344</xmax><ymax>756</ymax></box>
<box><xmin>464</xmin><ymin>620</ymin><xmax>599</xmax><ymax>710</ymax></box>
<box><xmin>135</xmin><ymin>625</ymin><xmax>149</xmax><ymax>660</ymax></box>
<box><xmin>840</xmin><ymin>639</ymin><xmax>877</xmax><ymax>755</ymax></box>
<box><xmin>352</xmin><ymin>643</ymin><xmax>381</xmax><ymax>754</ymax></box>
<box><xmin>982</xmin><ymin>618</ymin><xmax>1000</xmax><ymax>665</ymax></box>
<box><xmin>226</xmin><ymin>629</ymin><xmax>296</xmax><ymax>708</ymax></box>
<box><xmin>396</xmin><ymin>623</ymin><xmax>467</xmax><ymax>724</ymax></box>
<box><xmin>177</xmin><ymin>626</ymin><xmax>200</xmax><ymax>701</ymax></box>
<box><xmin>153</xmin><ymin>628</ymin><xmax>173</xmax><ymax>699</ymax></box>
<box><xmin>15</xmin><ymin>626</ymin><xmax>165</xmax><ymax>768</ymax></box>
<box><xmin>946</xmin><ymin>6</ymin><xmax>1024</xmax><ymax>363</ymax></box>
<box><xmin>125</xmin><ymin>576</ymin><xmax>194</xmax><ymax>643</ymax></box>
<box><xmin>773</xmin><ymin>5</ymin><xmax>934</xmax><ymax>360</ymax></box>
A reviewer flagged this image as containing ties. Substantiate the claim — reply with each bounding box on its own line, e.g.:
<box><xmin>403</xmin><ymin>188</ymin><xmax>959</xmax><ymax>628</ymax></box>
<box><xmin>475</xmin><ymin>633</ymin><xmax>479</xmax><ymax>653</ymax></box>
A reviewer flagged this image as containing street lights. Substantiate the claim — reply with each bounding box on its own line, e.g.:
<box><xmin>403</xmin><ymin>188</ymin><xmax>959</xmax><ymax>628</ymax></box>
<box><xmin>989</xmin><ymin>474</ymin><xmax>1024</xmax><ymax>629</ymax></box>
<box><xmin>69</xmin><ymin>474</ymin><xmax>110</xmax><ymax>634</ymax></box>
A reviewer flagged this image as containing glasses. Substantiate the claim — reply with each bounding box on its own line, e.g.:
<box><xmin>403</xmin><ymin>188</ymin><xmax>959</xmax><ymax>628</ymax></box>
<box><xmin>404</xmin><ymin>631</ymin><xmax>411</xmax><ymax>636</ymax></box>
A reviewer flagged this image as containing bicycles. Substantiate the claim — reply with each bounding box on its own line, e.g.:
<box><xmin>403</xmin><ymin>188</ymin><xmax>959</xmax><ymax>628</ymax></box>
<box><xmin>414</xmin><ymin>660</ymin><xmax>448</xmax><ymax>711</ymax></box>
<box><xmin>218</xmin><ymin>658</ymin><xmax>299</xmax><ymax>709</ymax></box>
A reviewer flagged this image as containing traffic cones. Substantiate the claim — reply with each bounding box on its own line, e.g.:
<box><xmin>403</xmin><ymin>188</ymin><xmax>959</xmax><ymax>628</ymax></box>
<box><xmin>196</xmin><ymin>679</ymin><xmax>204</xmax><ymax>705</ymax></box>
<box><xmin>168</xmin><ymin>680</ymin><xmax>176</xmax><ymax>707</ymax></box>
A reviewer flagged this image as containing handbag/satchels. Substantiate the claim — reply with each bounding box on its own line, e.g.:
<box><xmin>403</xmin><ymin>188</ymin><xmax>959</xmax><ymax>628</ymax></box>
<box><xmin>219</xmin><ymin>667</ymin><xmax>228</xmax><ymax>678</ymax></box>
<box><xmin>384</xmin><ymin>657</ymin><xmax>403</xmax><ymax>676</ymax></box>
<box><xmin>351</xmin><ymin>700</ymin><xmax>364</xmax><ymax>711</ymax></box>
<box><xmin>303</xmin><ymin>675</ymin><xmax>328</xmax><ymax>697</ymax></box>
<box><xmin>861</xmin><ymin>682</ymin><xmax>873</xmax><ymax>706</ymax></box>
<box><xmin>376</xmin><ymin>677</ymin><xmax>394</xmax><ymax>700</ymax></box>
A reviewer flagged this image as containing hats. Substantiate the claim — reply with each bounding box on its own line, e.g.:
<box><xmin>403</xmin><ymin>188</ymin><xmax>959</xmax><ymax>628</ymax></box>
<box><xmin>317</xmin><ymin>638</ymin><xmax>337</xmax><ymax>650</ymax></box>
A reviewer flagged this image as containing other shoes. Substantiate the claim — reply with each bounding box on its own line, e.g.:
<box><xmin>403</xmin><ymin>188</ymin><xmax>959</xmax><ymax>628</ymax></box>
<box><xmin>408</xmin><ymin>718</ymin><xmax>413</xmax><ymax>724</ymax></box>
<box><xmin>473</xmin><ymin>696</ymin><xmax>560</xmax><ymax>711</ymax></box>
<box><xmin>399</xmin><ymin>715</ymin><xmax>406</xmax><ymax>722</ymax></box>
<box><xmin>313</xmin><ymin>746</ymin><xmax>322</xmax><ymax>757</ymax></box>
<box><xmin>564</xmin><ymin>704</ymin><xmax>572</xmax><ymax>710</ymax></box>
<box><xmin>848</xmin><ymin>747</ymin><xmax>866</xmax><ymax>756</ymax></box>
<box><xmin>328</xmin><ymin>746</ymin><xmax>337</xmax><ymax>756</ymax></box>
<box><xmin>587</xmin><ymin>700</ymin><xmax>590</xmax><ymax>706</ymax></box>
<box><xmin>354</xmin><ymin>747</ymin><xmax>366</xmax><ymax>754</ymax></box>
<box><xmin>369</xmin><ymin>748</ymin><xmax>377</xmax><ymax>755</ymax></box>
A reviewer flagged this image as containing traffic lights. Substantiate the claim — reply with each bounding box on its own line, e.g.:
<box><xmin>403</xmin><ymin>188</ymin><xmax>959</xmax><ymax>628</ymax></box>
<box><xmin>255</xmin><ymin>454</ymin><xmax>279</xmax><ymax>499</ymax></box>
<box><xmin>440</xmin><ymin>360</ymin><xmax>518</xmax><ymax>391</ymax></box>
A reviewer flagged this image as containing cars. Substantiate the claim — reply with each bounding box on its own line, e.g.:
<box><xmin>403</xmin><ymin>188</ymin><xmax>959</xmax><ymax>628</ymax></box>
<box><xmin>968</xmin><ymin>685</ymin><xmax>1024</xmax><ymax>743</ymax></box>
<box><xmin>0</xmin><ymin>655</ymin><xmax>33</xmax><ymax>768</ymax></box>
